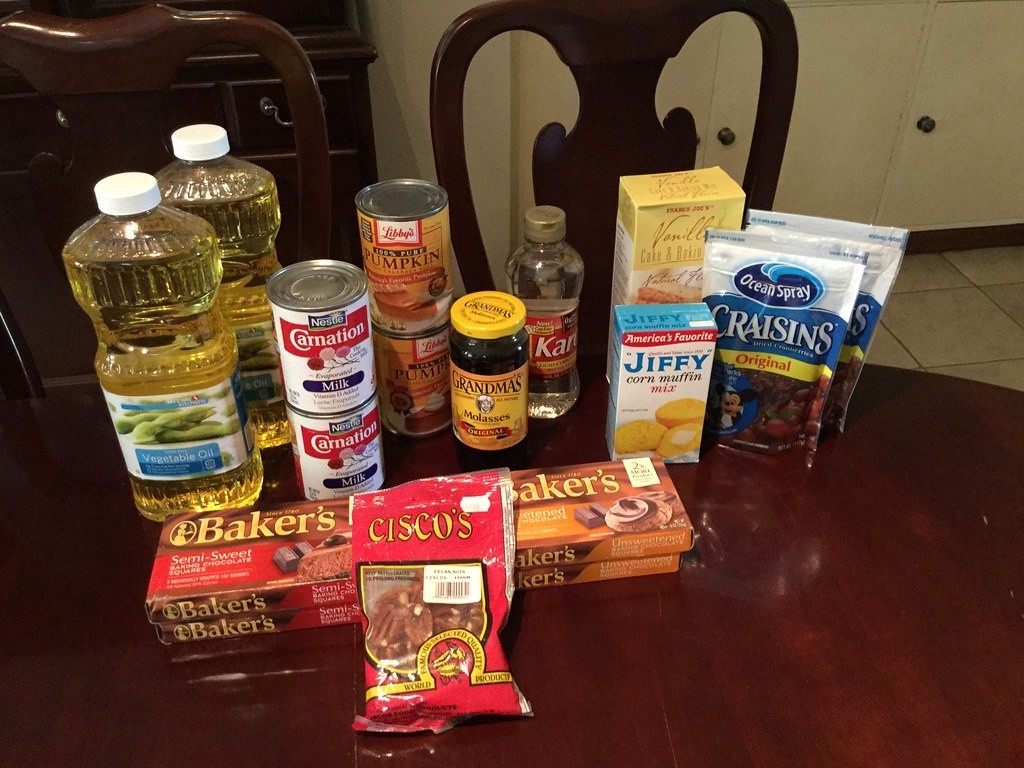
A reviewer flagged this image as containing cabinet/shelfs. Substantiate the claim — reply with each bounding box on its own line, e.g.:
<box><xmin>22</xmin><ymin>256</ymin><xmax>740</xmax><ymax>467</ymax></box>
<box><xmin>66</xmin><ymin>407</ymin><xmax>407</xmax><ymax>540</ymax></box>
<box><xmin>0</xmin><ymin>0</ymin><xmax>379</xmax><ymax>313</ymax></box>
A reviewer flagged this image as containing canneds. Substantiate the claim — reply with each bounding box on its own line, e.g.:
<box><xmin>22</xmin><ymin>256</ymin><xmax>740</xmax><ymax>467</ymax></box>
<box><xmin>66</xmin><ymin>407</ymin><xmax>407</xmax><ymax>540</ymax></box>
<box><xmin>264</xmin><ymin>175</ymin><xmax>530</xmax><ymax>504</ymax></box>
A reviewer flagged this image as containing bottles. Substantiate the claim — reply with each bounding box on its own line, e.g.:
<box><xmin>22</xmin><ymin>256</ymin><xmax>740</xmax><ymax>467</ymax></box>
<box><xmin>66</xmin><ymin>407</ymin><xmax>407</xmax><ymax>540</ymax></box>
<box><xmin>62</xmin><ymin>172</ymin><xmax>263</xmax><ymax>521</ymax></box>
<box><xmin>154</xmin><ymin>124</ymin><xmax>292</xmax><ymax>450</ymax></box>
<box><xmin>505</xmin><ymin>205</ymin><xmax>585</xmax><ymax>419</ymax></box>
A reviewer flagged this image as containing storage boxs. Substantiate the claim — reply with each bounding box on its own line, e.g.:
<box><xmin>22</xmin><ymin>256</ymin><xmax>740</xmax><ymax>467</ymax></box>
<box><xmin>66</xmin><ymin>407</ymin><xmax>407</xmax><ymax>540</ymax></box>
<box><xmin>143</xmin><ymin>498</ymin><xmax>363</xmax><ymax>647</ymax></box>
<box><xmin>512</xmin><ymin>455</ymin><xmax>695</xmax><ymax>589</ymax></box>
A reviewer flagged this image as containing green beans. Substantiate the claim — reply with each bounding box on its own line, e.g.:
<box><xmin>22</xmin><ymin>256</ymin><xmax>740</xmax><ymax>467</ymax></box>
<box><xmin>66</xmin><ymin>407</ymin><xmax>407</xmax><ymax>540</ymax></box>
<box><xmin>108</xmin><ymin>384</ymin><xmax>243</xmax><ymax>445</ymax></box>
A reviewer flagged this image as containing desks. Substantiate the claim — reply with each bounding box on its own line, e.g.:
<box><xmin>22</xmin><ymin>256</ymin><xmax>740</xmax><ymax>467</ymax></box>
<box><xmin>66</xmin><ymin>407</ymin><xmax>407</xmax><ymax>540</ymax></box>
<box><xmin>0</xmin><ymin>352</ymin><xmax>1024</xmax><ymax>768</ymax></box>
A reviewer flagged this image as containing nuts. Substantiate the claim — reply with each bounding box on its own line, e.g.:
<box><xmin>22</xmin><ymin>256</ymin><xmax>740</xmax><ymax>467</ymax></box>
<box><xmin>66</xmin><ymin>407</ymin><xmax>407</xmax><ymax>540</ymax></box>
<box><xmin>362</xmin><ymin>575</ymin><xmax>483</xmax><ymax>660</ymax></box>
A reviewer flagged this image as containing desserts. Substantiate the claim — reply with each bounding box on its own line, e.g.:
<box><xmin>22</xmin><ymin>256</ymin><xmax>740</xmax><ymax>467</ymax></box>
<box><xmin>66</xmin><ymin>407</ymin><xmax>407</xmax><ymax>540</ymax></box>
<box><xmin>613</xmin><ymin>398</ymin><xmax>706</xmax><ymax>459</ymax></box>
<box><xmin>573</xmin><ymin>489</ymin><xmax>685</xmax><ymax>534</ymax></box>
<box><xmin>273</xmin><ymin>526</ymin><xmax>354</xmax><ymax>583</ymax></box>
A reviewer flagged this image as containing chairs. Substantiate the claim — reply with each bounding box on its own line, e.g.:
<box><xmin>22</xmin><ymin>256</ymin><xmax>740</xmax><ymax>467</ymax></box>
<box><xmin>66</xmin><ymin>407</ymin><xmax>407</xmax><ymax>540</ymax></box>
<box><xmin>429</xmin><ymin>0</ymin><xmax>798</xmax><ymax>363</ymax></box>
<box><xmin>0</xmin><ymin>2</ymin><xmax>332</xmax><ymax>399</ymax></box>
